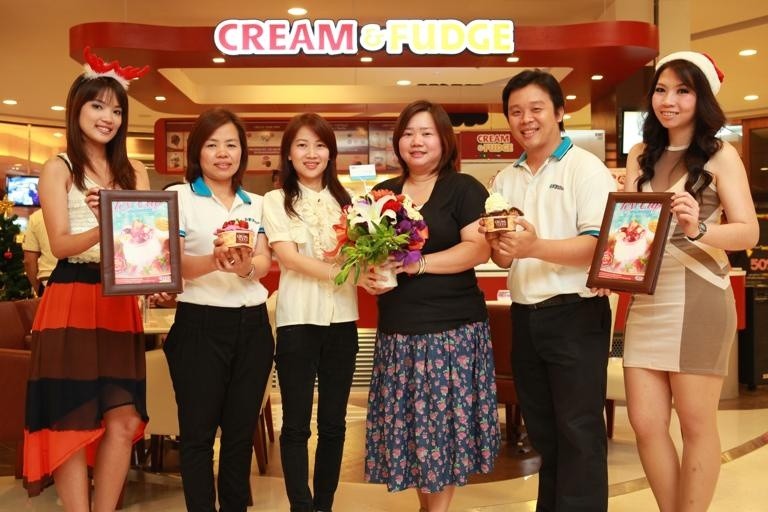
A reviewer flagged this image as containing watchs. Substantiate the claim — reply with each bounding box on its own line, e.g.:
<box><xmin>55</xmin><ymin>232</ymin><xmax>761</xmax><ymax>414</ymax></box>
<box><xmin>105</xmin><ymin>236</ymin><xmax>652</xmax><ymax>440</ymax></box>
<box><xmin>687</xmin><ymin>222</ymin><xmax>707</xmax><ymax>241</ymax></box>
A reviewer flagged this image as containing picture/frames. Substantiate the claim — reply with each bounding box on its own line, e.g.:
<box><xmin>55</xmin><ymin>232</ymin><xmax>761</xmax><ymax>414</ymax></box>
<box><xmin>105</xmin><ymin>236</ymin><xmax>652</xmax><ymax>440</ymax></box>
<box><xmin>95</xmin><ymin>186</ymin><xmax>183</xmax><ymax>298</ymax></box>
<box><xmin>584</xmin><ymin>190</ymin><xmax>677</xmax><ymax>299</ymax></box>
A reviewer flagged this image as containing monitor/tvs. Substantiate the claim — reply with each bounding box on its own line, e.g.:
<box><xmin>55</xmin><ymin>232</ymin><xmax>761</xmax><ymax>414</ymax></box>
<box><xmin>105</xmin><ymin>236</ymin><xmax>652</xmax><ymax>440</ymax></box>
<box><xmin>617</xmin><ymin>107</ymin><xmax>650</xmax><ymax>157</ymax></box>
<box><xmin>5</xmin><ymin>174</ymin><xmax>43</xmax><ymax>207</ymax></box>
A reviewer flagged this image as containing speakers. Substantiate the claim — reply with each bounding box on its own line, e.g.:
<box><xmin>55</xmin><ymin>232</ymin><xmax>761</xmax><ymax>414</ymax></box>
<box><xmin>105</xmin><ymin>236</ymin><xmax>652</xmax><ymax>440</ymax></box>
<box><xmin>738</xmin><ymin>286</ymin><xmax>768</xmax><ymax>390</ymax></box>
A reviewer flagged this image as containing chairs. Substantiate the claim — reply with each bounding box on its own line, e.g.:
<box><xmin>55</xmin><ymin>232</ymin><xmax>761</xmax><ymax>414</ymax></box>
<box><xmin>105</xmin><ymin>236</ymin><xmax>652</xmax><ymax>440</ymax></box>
<box><xmin>0</xmin><ymin>293</ymin><xmax>632</xmax><ymax>481</ymax></box>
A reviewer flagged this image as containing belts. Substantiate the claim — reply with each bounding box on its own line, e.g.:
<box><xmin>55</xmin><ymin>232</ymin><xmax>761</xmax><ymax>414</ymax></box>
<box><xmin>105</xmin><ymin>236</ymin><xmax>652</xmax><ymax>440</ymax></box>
<box><xmin>516</xmin><ymin>292</ymin><xmax>608</xmax><ymax>311</ymax></box>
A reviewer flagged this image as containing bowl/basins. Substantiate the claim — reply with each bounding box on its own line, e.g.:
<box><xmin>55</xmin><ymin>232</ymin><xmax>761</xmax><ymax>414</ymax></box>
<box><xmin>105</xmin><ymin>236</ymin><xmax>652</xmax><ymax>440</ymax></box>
<box><xmin>214</xmin><ymin>230</ymin><xmax>255</xmax><ymax>251</ymax></box>
<box><xmin>482</xmin><ymin>214</ymin><xmax>518</xmax><ymax>235</ymax></box>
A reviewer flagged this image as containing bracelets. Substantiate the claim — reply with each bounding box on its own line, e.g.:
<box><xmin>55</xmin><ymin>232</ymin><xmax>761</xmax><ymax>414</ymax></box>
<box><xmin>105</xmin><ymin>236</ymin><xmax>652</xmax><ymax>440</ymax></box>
<box><xmin>237</xmin><ymin>264</ymin><xmax>256</xmax><ymax>281</ymax></box>
<box><xmin>419</xmin><ymin>255</ymin><xmax>428</xmax><ymax>274</ymax></box>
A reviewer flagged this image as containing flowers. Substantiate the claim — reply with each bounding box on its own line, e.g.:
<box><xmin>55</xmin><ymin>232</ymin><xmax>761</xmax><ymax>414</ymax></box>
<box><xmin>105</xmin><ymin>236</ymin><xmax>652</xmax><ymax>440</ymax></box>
<box><xmin>320</xmin><ymin>185</ymin><xmax>432</xmax><ymax>288</ymax></box>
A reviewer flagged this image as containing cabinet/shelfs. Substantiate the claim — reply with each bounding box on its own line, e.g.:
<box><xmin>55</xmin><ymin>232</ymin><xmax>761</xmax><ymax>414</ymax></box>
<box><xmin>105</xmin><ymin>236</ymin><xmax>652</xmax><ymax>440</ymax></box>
<box><xmin>738</xmin><ymin>116</ymin><xmax>768</xmax><ymax>259</ymax></box>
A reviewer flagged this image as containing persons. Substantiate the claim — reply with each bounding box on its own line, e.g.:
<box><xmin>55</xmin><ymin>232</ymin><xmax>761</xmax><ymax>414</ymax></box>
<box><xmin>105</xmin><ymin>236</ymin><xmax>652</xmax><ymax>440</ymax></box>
<box><xmin>478</xmin><ymin>69</ymin><xmax>617</xmax><ymax>512</ymax></box>
<box><xmin>588</xmin><ymin>52</ymin><xmax>761</xmax><ymax>511</ymax></box>
<box><xmin>14</xmin><ymin>182</ymin><xmax>39</xmax><ymax>206</ymax></box>
<box><xmin>357</xmin><ymin>99</ymin><xmax>501</xmax><ymax>512</ymax></box>
<box><xmin>261</xmin><ymin>112</ymin><xmax>390</xmax><ymax>512</ymax></box>
<box><xmin>163</xmin><ymin>109</ymin><xmax>275</xmax><ymax>512</ymax></box>
<box><xmin>21</xmin><ymin>206</ymin><xmax>59</xmax><ymax>298</ymax></box>
<box><xmin>15</xmin><ymin>72</ymin><xmax>171</xmax><ymax>512</ymax></box>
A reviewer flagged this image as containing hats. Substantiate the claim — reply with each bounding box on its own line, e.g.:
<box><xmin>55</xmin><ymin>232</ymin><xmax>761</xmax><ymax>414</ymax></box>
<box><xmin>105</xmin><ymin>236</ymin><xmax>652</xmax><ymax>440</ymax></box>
<box><xmin>655</xmin><ymin>51</ymin><xmax>724</xmax><ymax>96</ymax></box>
